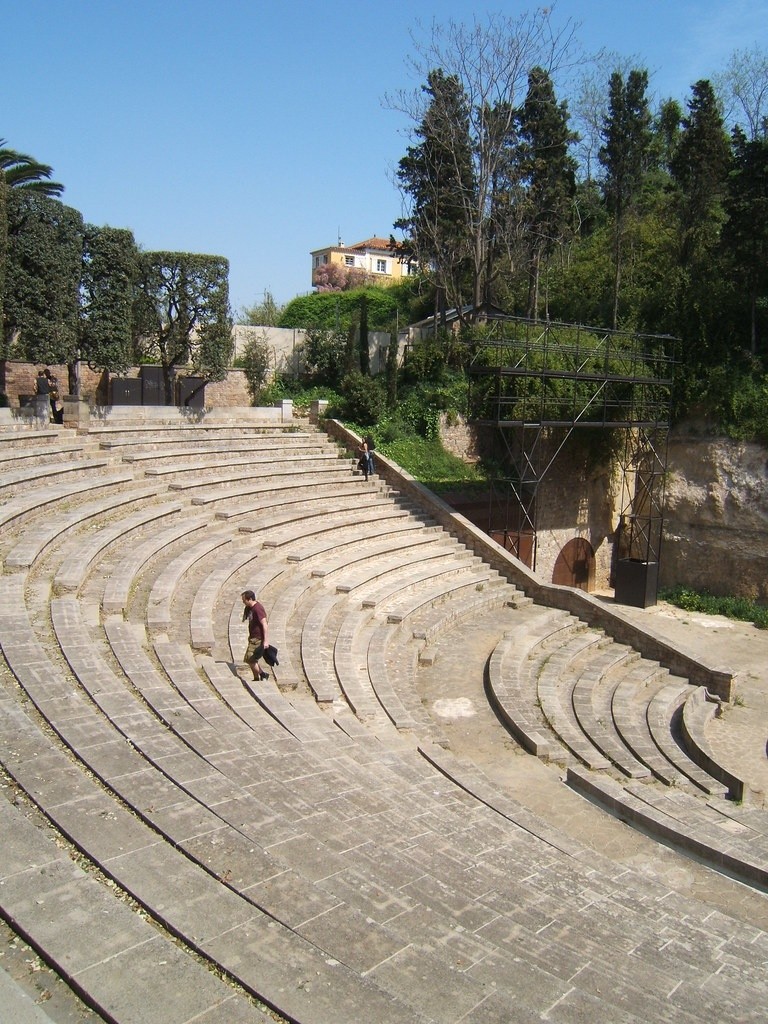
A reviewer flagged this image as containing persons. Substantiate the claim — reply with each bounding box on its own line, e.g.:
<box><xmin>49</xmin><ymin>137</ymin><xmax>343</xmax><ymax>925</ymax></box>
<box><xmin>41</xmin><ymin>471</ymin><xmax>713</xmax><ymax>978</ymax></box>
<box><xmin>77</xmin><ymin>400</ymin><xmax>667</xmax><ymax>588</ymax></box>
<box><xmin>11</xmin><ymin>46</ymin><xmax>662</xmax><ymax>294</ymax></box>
<box><xmin>365</xmin><ymin>433</ymin><xmax>374</xmax><ymax>475</ymax></box>
<box><xmin>241</xmin><ymin>590</ymin><xmax>269</xmax><ymax>681</ymax></box>
<box><xmin>357</xmin><ymin>437</ymin><xmax>368</xmax><ymax>481</ymax></box>
<box><xmin>33</xmin><ymin>369</ymin><xmax>62</xmax><ymax>424</ymax></box>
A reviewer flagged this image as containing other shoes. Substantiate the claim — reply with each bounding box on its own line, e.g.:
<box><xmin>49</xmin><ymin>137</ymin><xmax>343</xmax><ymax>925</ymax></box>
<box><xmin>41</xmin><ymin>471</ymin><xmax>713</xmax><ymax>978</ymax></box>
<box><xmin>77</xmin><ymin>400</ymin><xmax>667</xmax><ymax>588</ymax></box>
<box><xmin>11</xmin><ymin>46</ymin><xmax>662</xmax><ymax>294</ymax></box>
<box><xmin>261</xmin><ymin>673</ymin><xmax>269</xmax><ymax>681</ymax></box>
